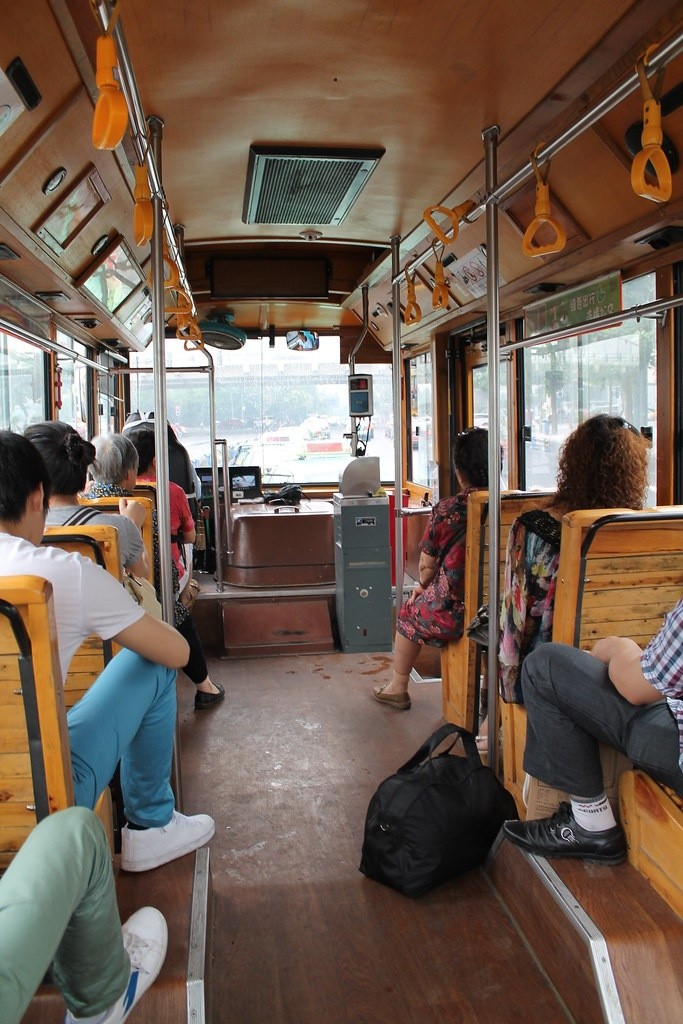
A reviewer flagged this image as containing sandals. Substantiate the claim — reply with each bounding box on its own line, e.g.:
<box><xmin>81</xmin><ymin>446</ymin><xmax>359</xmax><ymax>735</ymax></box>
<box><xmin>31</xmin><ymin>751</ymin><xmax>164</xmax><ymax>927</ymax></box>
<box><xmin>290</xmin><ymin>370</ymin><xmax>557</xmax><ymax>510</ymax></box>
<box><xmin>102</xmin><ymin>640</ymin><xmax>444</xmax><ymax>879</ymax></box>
<box><xmin>182</xmin><ymin>578</ymin><xmax>200</xmax><ymax>613</ymax></box>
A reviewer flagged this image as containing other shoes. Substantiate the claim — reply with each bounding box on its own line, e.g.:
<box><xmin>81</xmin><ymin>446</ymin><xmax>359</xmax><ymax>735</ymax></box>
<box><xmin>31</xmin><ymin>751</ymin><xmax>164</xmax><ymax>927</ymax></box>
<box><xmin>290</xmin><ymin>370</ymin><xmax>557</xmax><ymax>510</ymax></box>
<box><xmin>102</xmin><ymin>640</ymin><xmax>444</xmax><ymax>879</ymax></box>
<box><xmin>371</xmin><ymin>684</ymin><xmax>412</xmax><ymax>709</ymax></box>
<box><xmin>194</xmin><ymin>682</ymin><xmax>225</xmax><ymax>709</ymax></box>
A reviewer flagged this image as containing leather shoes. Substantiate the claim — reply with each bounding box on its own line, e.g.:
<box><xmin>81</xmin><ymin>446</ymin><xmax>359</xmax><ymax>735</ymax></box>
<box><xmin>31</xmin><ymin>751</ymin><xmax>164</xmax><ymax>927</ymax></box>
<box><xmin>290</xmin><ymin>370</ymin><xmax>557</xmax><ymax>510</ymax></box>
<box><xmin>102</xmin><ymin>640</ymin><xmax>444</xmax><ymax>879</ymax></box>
<box><xmin>502</xmin><ymin>800</ymin><xmax>626</xmax><ymax>865</ymax></box>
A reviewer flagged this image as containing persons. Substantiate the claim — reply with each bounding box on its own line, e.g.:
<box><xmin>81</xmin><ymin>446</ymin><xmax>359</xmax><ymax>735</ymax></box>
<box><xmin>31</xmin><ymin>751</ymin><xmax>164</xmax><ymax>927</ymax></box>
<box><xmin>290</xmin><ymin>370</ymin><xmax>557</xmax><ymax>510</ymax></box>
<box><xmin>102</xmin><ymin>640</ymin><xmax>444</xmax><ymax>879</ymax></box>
<box><xmin>295</xmin><ymin>331</ymin><xmax>313</xmax><ymax>350</ymax></box>
<box><xmin>188</xmin><ymin>462</ymin><xmax>202</xmax><ymax>500</ymax></box>
<box><xmin>0</xmin><ymin>806</ymin><xmax>169</xmax><ymax>1024</ymax></box>
<box><xmin>502</xmin><ymin>594</ymin><xmax>683</xmax><ymax>867</ymax></box>
<box><xmin>0</xmin><ymin>429</ymin><xmax>216</xmax><ymax>871</ymax></box>
<box><xmin>472</xmin><ymin>414</ymin><xmax>652</xmax><ymax>775</ymax></box>
<box><xmin>369</xmin><ymin>425</ymin><xmax>502</xmax><ymax>711</ymax></box>
<box><xmin>24</xmin><ymin>420</ymin><xmax>152</xmax><ymax>578</ymax></box>
<box><xmin>121</xmin><ymin>425</ymin><xmax>202</xmax><ymax>615</ymax></box>
<box><xmin>77</xmin><ymin>433</ymin><xmax>226</xmax><ymax>710</ymax></box>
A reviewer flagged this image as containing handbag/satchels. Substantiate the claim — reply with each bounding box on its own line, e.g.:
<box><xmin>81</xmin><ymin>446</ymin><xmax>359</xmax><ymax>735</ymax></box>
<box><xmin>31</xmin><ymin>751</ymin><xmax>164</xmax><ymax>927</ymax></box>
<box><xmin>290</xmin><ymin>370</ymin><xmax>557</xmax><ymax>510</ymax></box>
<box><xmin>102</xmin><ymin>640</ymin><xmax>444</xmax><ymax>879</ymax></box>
<box><xmin>121</xmin><ymin>571</ymin><xmax>163</xmax><ymax>620</ymax></box>
<box><xmin>263</xmin><ymin>485</ymin><xmax>311</xmax><ymax>506</ymax></box>
<box><xmin>466</xmin><ymin>604</ymin><xmax>504</xmax><ymax>647</ymax></box>
<box><xmin>357</xmin><ymin>723</ymin><xmax>521</xmax><ymax>896</ymax></box>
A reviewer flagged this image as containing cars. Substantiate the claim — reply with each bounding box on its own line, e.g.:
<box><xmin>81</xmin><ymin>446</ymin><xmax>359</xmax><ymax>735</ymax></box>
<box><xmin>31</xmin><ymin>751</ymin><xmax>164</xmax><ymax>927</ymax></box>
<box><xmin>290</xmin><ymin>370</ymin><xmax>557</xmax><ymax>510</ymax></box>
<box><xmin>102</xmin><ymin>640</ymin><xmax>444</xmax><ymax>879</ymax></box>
<box><xmin>304</xmin><ymin>413</ymin><xmax>431</xmax><ymax>449</ymax></box>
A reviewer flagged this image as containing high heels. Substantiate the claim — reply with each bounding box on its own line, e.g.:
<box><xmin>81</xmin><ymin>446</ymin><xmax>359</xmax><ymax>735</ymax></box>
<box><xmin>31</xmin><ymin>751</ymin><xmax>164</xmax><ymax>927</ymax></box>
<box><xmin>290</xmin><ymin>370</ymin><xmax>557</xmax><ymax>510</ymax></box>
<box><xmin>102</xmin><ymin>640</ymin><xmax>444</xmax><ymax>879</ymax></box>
<box><xmin>473</xmin><ymin>733</ymin><xmax>500</xmax><ymax>766</ymax></box>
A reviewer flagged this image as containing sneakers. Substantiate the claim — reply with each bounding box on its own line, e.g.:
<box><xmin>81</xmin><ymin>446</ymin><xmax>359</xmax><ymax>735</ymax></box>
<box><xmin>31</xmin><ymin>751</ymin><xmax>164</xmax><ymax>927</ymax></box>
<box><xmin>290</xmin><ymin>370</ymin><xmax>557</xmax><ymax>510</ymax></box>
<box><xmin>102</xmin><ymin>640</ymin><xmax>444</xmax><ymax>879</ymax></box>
<box><xmin>64</xmin><ymin>906</ymin><xmax>168</xmax><ymax>1024</ymax></box>
<box><xmin>121</xmin><ymin>809</ymin><xmax>215</xmax><ymax>872</ymax></box>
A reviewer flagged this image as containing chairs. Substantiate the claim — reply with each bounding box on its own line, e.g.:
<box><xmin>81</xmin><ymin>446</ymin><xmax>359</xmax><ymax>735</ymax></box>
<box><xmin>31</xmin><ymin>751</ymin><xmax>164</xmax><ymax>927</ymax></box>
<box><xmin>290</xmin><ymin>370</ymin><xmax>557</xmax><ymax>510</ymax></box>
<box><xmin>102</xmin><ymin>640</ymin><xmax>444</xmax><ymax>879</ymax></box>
<box><xmin>0</xmin><ymin>482</ymin><xmax>160</xmax><ymax>873</ymax></box>
<box><xmin>441</xmin><ymin>491</ymin><xmax>683</xmax><ymax>921</ymax></box>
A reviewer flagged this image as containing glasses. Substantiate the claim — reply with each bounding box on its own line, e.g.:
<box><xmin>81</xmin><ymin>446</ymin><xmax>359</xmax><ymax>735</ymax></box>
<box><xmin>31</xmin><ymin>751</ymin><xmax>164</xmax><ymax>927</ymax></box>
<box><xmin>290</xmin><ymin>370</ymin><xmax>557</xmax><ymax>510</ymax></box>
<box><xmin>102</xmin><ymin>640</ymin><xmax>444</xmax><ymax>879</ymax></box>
<box><xmin>614</xmin><ymin>417</ymin><xmax>639</xmax><ymax>436</ymax></box>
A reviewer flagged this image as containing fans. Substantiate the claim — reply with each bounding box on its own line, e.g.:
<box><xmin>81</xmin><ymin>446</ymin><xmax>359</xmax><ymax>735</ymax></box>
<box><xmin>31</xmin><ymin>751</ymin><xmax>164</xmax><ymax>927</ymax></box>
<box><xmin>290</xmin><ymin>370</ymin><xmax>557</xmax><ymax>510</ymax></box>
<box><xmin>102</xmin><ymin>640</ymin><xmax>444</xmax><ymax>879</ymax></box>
<box><xmin>197</xmin><ymin>309</ymin><xmax>246</xmax><ymax>350</ymax></box>
